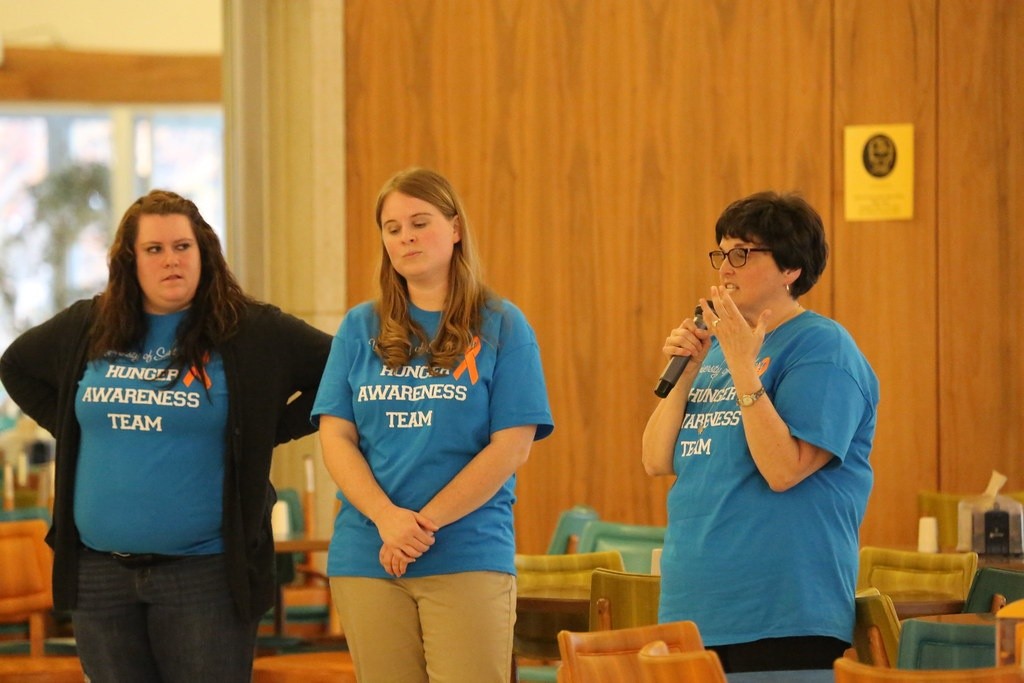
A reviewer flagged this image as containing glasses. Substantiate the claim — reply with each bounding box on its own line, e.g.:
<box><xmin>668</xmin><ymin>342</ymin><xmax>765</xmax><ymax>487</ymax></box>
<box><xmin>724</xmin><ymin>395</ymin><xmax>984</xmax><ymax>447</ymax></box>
<box><xmin>708</xmin><ymin>247</ymin><xmax>772</xmax><ymax>270</ymax></box>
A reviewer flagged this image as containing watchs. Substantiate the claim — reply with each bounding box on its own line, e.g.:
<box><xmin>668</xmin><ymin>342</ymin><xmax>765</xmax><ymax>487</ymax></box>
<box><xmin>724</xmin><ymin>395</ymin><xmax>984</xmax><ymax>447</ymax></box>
<box><xmin>736</xmin><ymin>386</ymin><xmax>765</xmax><ymax>407</ymax></box>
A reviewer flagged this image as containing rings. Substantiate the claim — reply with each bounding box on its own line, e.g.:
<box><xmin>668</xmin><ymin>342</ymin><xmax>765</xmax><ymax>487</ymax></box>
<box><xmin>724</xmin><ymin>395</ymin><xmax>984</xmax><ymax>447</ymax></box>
<box><xmin>712</xmin><ymin>319</ymin><xmax>721</xmax><ymax>327</ymax></box>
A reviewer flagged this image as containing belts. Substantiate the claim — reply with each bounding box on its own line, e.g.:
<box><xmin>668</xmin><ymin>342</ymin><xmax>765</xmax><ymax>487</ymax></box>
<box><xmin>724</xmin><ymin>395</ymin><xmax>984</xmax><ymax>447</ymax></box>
<box><xmin>81</xmin><ymin>544</ymin><xmax>156</xmax><ymax>565</ymax></box>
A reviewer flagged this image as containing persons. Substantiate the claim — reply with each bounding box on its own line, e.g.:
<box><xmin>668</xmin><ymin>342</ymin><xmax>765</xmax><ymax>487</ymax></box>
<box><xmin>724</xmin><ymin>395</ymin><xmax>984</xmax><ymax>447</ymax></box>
<box><xmin>641</xmin><ymin>192</ymin><xmax>880</xmax><ymax>683</ymax></box>
<box><xmin>309</xmin><ymin>168</ymin><xmax>554</xmax><ymax>683</ymax></box>
<box><xmin>0</xmin><ymin>190</ymin><xmax>334</xmax><ymax>683</ymax></box>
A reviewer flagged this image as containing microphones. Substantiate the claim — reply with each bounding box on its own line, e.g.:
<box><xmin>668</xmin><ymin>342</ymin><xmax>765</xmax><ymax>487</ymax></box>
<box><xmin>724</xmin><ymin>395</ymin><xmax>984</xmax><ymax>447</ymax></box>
<box><xmin>653</xmin><ymin>300</ymin><xmax>718</xmax><ymax>398</ymax></box>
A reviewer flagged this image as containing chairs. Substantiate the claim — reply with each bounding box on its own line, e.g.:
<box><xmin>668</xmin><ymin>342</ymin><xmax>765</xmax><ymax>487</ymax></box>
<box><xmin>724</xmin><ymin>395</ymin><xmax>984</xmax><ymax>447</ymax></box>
<box><xmin>0</xmin><ymin>485</ymin><xmax>1024</xmax><ymax>683</ymax></box>
